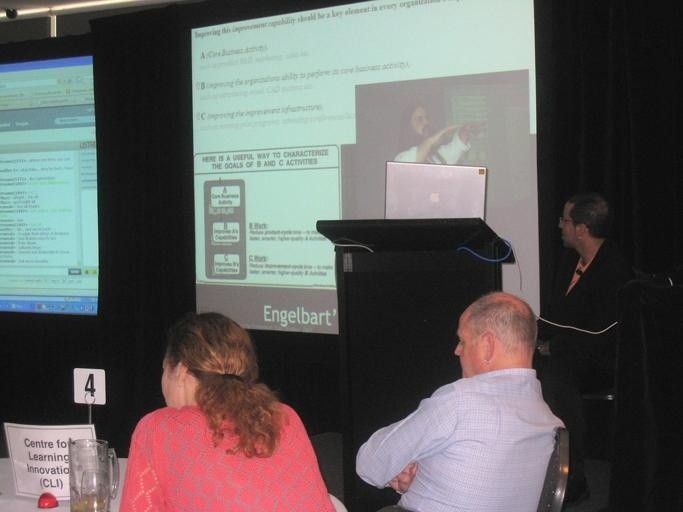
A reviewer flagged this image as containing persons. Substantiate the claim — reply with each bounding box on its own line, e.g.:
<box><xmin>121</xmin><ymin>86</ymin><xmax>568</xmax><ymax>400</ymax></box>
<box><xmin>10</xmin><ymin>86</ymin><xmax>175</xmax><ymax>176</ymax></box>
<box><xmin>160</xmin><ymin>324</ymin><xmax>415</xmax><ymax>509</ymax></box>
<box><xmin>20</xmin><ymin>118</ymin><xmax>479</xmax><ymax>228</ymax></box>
<box><xmin>353</xmin><ymin>290</ymin><xmax>573</xmax><ymax>512</ymax></box>
<box><xmin>535</xmin><ymin>191</ymin><xmax>661</xmax><ymax>512</ymax></box>
<box><xmin>116</xmin><ymin>311</ymin><xmax>340</xmax><ymax>512</ymax></box>
<box><xmin>392</xmin><ymin>98</ymin><xmax>485</xmax><ymax>168</ymax></box>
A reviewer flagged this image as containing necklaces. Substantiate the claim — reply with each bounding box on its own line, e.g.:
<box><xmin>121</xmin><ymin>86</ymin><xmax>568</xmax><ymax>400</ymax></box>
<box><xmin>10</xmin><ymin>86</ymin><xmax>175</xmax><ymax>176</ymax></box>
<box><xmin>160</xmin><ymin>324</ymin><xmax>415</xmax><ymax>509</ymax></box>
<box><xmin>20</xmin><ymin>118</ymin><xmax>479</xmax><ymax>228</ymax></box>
<box><xmin>574</xmin><ymin>253</ymin><xmax>590</xmax><ymax>277</ymax></box>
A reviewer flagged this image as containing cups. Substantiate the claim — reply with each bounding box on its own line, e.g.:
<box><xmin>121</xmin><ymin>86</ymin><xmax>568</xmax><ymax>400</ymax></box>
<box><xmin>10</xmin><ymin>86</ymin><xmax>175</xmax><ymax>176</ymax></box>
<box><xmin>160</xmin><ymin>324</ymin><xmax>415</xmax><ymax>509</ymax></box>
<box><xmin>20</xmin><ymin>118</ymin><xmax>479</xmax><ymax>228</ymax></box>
<box><xmin>69</xmin><ymin>438</ymin><xmax>119</xmax><ymax>511</ymax></box>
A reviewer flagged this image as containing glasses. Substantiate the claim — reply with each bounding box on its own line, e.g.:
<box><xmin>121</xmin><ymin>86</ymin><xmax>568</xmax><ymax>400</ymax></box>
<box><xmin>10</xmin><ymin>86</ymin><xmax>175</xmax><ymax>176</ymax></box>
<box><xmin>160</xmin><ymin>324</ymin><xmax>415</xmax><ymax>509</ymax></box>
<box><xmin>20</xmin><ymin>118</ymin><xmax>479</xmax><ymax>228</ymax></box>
<box><xmin>559</xmin><ymin>216</ymin><xmax>574</xmax><ymax>224</ymax></box>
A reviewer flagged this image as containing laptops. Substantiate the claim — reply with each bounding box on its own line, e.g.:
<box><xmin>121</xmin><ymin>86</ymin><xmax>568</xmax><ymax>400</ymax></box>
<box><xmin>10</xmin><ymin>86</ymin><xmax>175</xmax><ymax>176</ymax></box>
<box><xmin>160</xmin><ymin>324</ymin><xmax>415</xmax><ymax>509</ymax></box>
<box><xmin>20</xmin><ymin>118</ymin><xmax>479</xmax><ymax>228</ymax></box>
<box><xmin>381</xmin><ymin>158</ymin><xmax>487</xmax><ymax>226</ymax></box>
